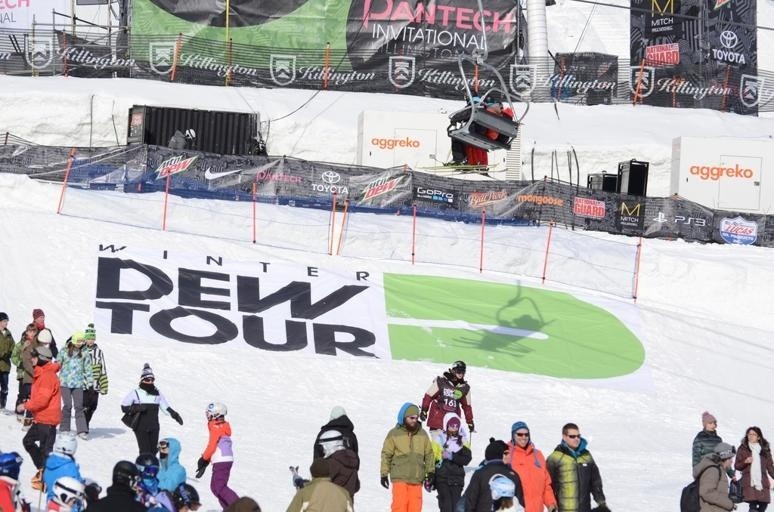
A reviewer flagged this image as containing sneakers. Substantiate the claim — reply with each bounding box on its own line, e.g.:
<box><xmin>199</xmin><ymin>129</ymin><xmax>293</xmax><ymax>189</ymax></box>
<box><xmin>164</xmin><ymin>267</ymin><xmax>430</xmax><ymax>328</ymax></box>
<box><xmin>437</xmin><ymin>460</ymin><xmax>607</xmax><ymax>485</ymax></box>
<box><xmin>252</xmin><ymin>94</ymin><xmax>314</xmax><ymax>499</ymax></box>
<box><xmin>17</xmin><ymin>414</ymin><xmax>32</xmax><ymax>431</ymax></box>
<box><xmin>78</xmin><ymin>432</ymin><xmax>87</xmax><ymax>439</ymax></box>
<box><xmin>31</xmin><ymin>470</ymin><xmax>49</xmax><ymax>492</ymax></box>
<box><xmin>60</xmin><ymin>431</ymin><xmax>70</xmax><ymax>440</ymax></box>
<box><xmin>0</xmin><ymin>407</ymin><xmax>13</xmax><ymax>416</ymax></box>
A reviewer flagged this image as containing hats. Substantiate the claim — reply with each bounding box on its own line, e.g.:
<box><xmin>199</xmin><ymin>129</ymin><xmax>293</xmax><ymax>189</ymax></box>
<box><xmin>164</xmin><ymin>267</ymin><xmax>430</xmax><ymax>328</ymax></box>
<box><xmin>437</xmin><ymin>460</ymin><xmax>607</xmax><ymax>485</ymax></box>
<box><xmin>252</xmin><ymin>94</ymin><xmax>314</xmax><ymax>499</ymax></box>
<box><xmin>173</xmin><ymin>482</ymin><xmax>199</xmax><ymax>510</ymax></box>
<box><xmin>310</xmin><ymin>458</ymin><xmax>329</xmax><ymax>477</ymax></box>
<box><xmin>84</xmin><ymin>323</ymin><xmax>96</xmax><ymax>340</ymax></box>
<box><xmin>330</xmin><ymin>406</ymin><xmax>346</xmax><ymax>420</ymax></box>
<box><xmin>38</xmin><ymin>329</ymin><xmax>52</xmax><ymax>343</ymax></box>
<box><xmin>72</xmin><ymin>332</ymin><xmax>85</xmax><ymax>345</ymax></box>
<box><xmin>33</xmin><ymin>309</ymin><xmax>45</xmax><ymax>319</ymax></box>
<box><xmin>0</xmin><ymin>311</ymin><xmax>9</xmax><ymax>322</ymax></box>
<box><xmin>444</xmin><ymin>412</ymin><xmax>460</xmax><ymax>424</ymax></box>
<box><xmin>401</xmin><ymin>403</ymin><xmax>419</xmax><ymax>417</ymax></box>
<box><xmin>141</xmin><ymin>363</ymin><xmax>154</xmax><ymax>380</ymax></box>
<box><xmin>703</xmin><ymin>412</ymin><xmax>716</xmax><ymax>426</ymax></box>
<box><xmin>31</xmin><ymin>347</ymin><xmax>52</xmax><ymax>362</ymax></box>
<box><xmin>485</xmin><ymin>438</ymin><xmax>509</xmax><ymax>460</ymax></box>
<box><xmin>512</xmin><ymin>422</ymin><xmax>529</xmax><ymax>432</ymax></box>
<box><xmin>713</xmin><ymin>443</ymin><xmax>736</xmax><ymax>459</ymax></box>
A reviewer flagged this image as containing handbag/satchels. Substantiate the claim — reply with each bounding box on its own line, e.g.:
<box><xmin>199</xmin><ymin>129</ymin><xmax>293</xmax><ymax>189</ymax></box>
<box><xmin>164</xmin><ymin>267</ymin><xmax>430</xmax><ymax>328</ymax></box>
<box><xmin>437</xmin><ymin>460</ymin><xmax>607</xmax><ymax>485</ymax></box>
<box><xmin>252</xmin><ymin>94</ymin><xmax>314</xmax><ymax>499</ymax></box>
<box><xmin>121</xmin><ymin>403</ymin><xmax>141</xmax><ymax>430</ymax></box>
<box><xmin>729</xmin><ymin>480</ymin><xmax>743</xmax><ymax>503</ymax></box>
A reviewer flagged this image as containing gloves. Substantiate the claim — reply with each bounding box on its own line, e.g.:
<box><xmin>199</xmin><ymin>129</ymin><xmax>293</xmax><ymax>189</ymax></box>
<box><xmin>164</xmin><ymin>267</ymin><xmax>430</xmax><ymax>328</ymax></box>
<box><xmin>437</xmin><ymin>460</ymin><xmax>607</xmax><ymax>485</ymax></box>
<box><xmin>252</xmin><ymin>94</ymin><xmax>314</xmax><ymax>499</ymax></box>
<box><xmin>381</xmin><ymin>476</ymin><xmax>389</xmax><ymax>489</ymax></box>
<box><xmin>173</xmin><ymin>412</ymin><xmax>183</xmax><ymax>425</ymax></box>
<box><xmin>2</xmin><ymin>351</ymin><xmax>11</xmax><ymax>364</ymax></box>
<box><xmin>468</xmin><ymin>423</ymin><xmax>475</xmax><ymax>433</ymax></box>
<box><xmin>196</xmin><ymin>457</ymin><xmax>207</xmax><ymax>472</ymax></box>
<box><xmin>134</xmin><ymin>404</ymin><xmax>146</xmax><ymax>412</ymax></box>
<box><xmin>420</xmin><ymin>410</ymin><xmax>428</xmax><ymax>421</ymax></box>
<box><xmin>428</xmin><ymin>474</ymin><xmax>436</xmax><ymax>490</ymax></box>
<box><xmin>195</xmin><ymin>461</ymin><xmax>209</xmax><ymax>478</ymax></box>
<box><xmin>425</xmin><ymin>479</ymin><xmax>431</xmax><ymax>493</ymax></box>
<box><xmin>442</xmin><ymin>450</ymin><xmax>452</xmax><ymax>460</ymax></box>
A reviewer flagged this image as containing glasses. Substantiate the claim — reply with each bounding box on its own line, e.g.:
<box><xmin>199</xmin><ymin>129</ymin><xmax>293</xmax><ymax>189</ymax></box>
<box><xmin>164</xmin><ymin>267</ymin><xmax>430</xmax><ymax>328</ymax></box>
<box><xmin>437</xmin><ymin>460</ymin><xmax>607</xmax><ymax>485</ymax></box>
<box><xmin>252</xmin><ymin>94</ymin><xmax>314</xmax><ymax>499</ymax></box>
<box><xmin>455</xmin><ymin>370</ymin><xmax>465</xmax><ymax>374</ymax></box>
<box><xmin>515</xmin><ymin>433</ymin><xmax>529</xmax><ymax>436</ymax></box>
<box><xmin>142</xmin><ymin>378</ymin><xmax>154</xmax><ymax>382</ymax></box>
<box><xmin>567</xmin><ymin>434</ymin><xmax>580</xmax><ymax>439</ymax></box>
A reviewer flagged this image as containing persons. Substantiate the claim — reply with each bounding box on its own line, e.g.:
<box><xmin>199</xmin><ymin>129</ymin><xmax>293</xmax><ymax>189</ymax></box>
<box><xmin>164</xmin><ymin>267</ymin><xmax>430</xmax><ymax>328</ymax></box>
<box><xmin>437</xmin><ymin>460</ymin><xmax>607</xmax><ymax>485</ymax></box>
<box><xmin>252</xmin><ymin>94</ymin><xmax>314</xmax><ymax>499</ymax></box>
<box><xmin>0</xmin><ymin>308</ymin><xmax>262</xmax><ymax>512</ymax></box>
<box><xmin>691</xmin><ymin>410</ymin><xmax>737</xmax><ymax>484</ymax></box>
<box><xmin>284</xmin><ymin>457</ymin><xmax>354</xmax><ymax>511</ymax></box>
<box><xmin>167</xmin><ymin>128</ymin><xmax>197</xmax><ymax>149</ymax></box>
<box><xmin>691</xmin><ymin>442</ymin><xmax>739</xmax><ymax>511</ymax></box>
<box><xmin>446</xmin><ymin>90</ymin><xmax>514</xmax><ymax>166</ymax></box>
<box><xmin>312</xmin><ymin>405</ymin><xmax>360</xmax><ymax>472</ymax></box>
<box><xmin>289</xmin><ymin>430</ymin><xmax>361</xmax><ymax>498</ymax></box>
<box><xmin>733</xmin><ymin>425</ymin><xmax>774</xmax><ymax>512</ymax></box>
<box><xmin>378</xmin><ymin>401</ymin><xmax>611</xmax><ymax>512</ymax></box>
<box><xmin>417</xmin><ymin>359</ymin><xmax>473</xmax><ymax>449</ymax></box>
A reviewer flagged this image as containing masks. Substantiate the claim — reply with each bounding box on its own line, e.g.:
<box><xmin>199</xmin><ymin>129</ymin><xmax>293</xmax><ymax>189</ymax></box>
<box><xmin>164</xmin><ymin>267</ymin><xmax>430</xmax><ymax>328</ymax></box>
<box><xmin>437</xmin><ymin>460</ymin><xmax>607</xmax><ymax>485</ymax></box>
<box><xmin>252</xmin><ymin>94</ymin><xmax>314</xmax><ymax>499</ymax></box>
<box><xmin>160</xmin><ymin>452</ymin><xmax>168</xmax><ymax>459</ymax></box>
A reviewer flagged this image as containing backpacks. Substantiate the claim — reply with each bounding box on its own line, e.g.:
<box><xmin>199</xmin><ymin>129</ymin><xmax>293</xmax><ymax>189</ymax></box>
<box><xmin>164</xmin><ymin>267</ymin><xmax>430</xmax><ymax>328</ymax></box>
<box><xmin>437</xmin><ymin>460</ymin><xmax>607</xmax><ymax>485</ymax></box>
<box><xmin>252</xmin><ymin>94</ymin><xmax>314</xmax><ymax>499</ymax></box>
<box><xmin>681</xmin><ymin>465</ymin><xmax>721</xmax><ymax>512</ymax></box>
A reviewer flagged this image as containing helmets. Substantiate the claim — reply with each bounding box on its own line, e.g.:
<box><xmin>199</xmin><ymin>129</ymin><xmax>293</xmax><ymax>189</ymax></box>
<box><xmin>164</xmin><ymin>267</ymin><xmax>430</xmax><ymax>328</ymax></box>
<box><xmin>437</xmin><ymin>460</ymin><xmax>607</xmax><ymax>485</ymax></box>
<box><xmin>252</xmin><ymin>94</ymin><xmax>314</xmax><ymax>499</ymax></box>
<box><xmin>0</xmin><ymin>452</ymin><xmax>23</xmax><ymax>480</ymax></box>
<box><xmin>490</xmin><ymin>477</ymin><xmax>516</xmax><ymax>500</ymax></box>
<box><xmin>452</xmin><ymin>361</ymin><xmax>466</xmax><ymax>369</ymax></box>
<box><xmin>318</xmin><ymin>430</ymin><xmax>343</xmax><ymax>459</ymax></box>
<box><xmin>52</xmin><ymin>477</ymin><xmax>86</xmax><ymax>504</ymax></box>
<box><xmin>206</xmin><ymin>401</ymin><xmax>227</xmax><ymax>421</ymax></box>
<box><xmin>113</xmin><ymin>461</ymin><xmax>142</xmax><ymax>493</ymax></box>
<box><xmin>55</xmin><ymin>431</ymin><xmax>77</xmax><ymax>455</ymax></box>
